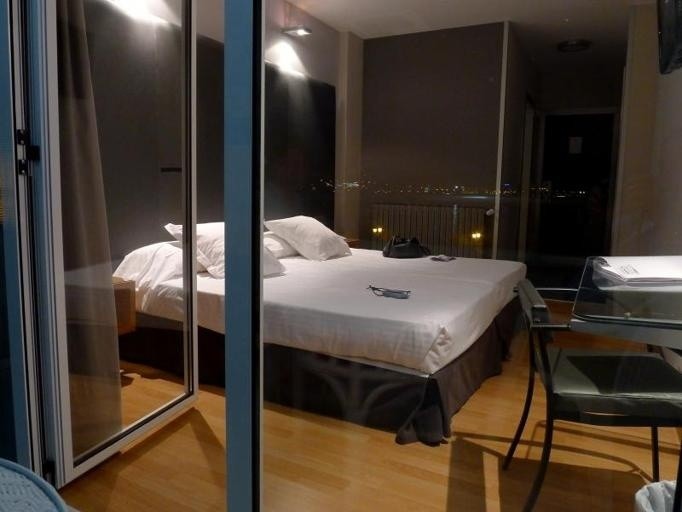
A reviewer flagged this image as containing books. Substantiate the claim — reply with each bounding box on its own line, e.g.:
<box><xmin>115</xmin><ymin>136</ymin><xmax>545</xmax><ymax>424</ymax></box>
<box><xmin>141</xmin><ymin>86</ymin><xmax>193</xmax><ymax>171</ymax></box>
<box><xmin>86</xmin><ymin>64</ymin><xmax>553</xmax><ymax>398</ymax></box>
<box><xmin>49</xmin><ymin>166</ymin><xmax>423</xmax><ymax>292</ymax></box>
<box><xmin>591</xmin><ymin>254</ymin><xmax>682</xmax><ymax>286</ymax></box>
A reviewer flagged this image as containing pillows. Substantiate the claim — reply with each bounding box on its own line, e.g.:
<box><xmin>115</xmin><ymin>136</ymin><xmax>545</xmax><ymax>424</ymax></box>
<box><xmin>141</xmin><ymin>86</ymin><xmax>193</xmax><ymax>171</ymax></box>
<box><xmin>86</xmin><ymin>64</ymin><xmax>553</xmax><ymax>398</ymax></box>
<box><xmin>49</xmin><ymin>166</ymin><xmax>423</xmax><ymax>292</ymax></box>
<box><xmin>267</xmin><ymin>216</ymin><xmax>349</xmax><ymax>260</ymax></box>
<box><xmin>163</xmin><ymin>222</ymin><xmax>285</xmax><ymax>279</ymax></box>
<box><xmin>116</xmin><ymin>232</ymin><xmax>295</xmax><ymax>282</ymax></box>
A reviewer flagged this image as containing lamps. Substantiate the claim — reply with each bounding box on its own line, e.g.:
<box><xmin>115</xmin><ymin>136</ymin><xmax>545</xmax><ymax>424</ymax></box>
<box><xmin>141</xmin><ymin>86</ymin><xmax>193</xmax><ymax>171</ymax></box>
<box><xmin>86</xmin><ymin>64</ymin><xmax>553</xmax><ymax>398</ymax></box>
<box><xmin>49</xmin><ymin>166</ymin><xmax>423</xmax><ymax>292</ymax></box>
<box><xmin>282</xmin><ymin>25</ymin><xmax>312</xmax><ymax>36</ymax></box>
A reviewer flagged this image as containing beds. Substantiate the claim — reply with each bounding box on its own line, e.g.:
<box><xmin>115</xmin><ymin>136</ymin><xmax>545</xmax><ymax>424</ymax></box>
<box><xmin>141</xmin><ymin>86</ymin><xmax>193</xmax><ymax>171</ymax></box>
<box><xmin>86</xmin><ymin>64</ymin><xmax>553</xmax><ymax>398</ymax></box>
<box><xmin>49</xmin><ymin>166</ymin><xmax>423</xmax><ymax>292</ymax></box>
<box><xmin>115</xmin><ymin>230</ymin><xmax>527</xmax><ymax>444</ymax></box>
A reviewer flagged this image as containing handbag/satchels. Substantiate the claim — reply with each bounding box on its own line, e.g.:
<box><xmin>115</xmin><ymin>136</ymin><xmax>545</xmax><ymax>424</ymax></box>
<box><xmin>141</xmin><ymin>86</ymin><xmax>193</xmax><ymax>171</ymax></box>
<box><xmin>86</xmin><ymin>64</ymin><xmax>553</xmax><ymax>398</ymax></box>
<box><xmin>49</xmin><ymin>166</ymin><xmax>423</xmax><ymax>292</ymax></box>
<box><xmin>383</xmin><ymin>234</ymin><xmax>430</xmax><ymax>258</ymax></box>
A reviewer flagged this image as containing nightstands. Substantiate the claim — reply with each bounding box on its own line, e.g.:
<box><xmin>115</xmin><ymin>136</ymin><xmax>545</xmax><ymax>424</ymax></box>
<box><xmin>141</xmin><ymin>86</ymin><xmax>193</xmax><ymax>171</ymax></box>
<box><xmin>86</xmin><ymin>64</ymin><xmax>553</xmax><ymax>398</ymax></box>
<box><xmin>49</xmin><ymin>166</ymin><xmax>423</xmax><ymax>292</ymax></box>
<box><xmin>344</xmin><ymin>238</ymin><xmax>361</xmax><ymax>248</ymax></box>
<box><xmin>62</xmin><ymin>275</ymin><xmax>137</xmax><ymax>372</ymax></box>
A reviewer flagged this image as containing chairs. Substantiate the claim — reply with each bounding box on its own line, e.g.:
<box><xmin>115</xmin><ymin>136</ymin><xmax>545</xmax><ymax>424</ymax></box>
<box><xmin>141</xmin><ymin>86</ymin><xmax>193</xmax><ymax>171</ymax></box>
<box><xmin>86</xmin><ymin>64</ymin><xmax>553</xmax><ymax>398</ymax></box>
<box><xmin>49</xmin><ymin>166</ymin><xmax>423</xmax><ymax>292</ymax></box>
<box><xmin>502</xmin><ymin>278</ymin><xmax>681</xmax><ymax>512</ymax></box>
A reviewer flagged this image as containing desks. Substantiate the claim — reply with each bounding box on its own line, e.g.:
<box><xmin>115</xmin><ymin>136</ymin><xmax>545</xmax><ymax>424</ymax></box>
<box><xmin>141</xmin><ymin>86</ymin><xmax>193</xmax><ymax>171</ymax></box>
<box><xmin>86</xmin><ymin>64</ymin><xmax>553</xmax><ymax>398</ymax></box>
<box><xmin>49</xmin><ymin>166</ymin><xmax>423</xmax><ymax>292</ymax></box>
<box><xmin>571</xmin><ymin>255</ymin><xmax>682</xmax><ymax>512</ymax></box>
